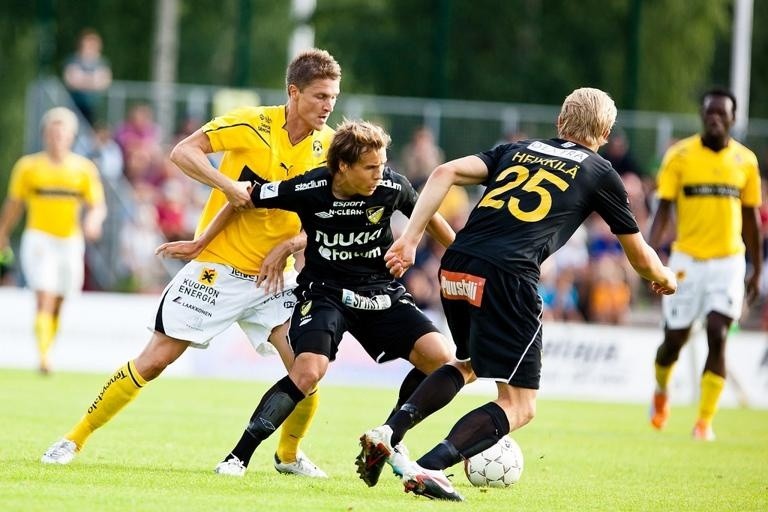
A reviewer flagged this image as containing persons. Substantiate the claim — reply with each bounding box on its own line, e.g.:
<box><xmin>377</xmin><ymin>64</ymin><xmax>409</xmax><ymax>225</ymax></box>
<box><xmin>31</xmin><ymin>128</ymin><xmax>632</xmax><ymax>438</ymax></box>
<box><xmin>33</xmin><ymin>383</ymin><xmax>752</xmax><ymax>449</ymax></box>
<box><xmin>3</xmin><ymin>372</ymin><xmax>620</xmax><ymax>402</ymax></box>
<box><xmin>155</xmin><ymin>116</ymin><xmax>457</xmax><ymax>476</ymax></box>
<box><xmin>391</xmin><ymin>130</ymin><xmax>469</xmax><ymax>305</ymax></box>
<box><xmin>643</xmin><ymin>87</ymin><xmax>762</xmax><ymax>444</ymax></box>
<box><xmin>2</xmin><ymin>31</ymin><xmax>219</xmax><ymax>375</ymax></box>
<box><xmin>538</xmin><ymin>124</ymin><xmax>768</xmax><ymax>327</ymax></box>
<box><xmin>357</xmin><ymin>84</ymin><xmax>680</xmax><ymax>502</ymax></box>
<box><xmin>37</xmin><ymin>49</ymin><xmax>337</xmax><ymax>480</ymax></box>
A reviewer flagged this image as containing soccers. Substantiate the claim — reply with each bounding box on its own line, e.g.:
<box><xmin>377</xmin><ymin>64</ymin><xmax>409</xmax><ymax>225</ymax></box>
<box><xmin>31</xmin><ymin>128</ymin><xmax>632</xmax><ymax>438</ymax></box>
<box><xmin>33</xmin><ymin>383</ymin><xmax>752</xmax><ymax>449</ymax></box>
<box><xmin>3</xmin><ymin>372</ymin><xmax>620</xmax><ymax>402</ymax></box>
<box><xmin>464</xmin><ymin>435</ymin><xmax>524</xmax><ymax>488</ymax></box>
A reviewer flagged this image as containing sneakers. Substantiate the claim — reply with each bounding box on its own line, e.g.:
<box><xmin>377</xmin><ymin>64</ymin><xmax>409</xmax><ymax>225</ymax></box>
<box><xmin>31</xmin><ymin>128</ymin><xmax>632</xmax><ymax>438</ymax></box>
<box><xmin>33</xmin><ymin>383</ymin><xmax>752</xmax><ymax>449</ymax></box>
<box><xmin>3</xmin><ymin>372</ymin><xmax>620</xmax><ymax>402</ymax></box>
<box><xmin>42</xmin><ymin>440</ymin><xmax>76</xmax><ymax>465</ymax></box>
<box><xmin>651</xmin><ymin>389</ymin><xmax>665</xmax><ymax>428</ymax></box>
<box><xmin>214</xmin><ymin>453</ymin><xmax>247</xmax><ymax>476</ymax></box>
<box><xmin>694</xmin><ymin>423</ymin><xmax>714</xmax><ymax>445</ymax></box>
<box><xmin>356</xmin><ymin>425</ymin><xmax>463</xmax><ymax>501</ymax></box>
<box><xmin>275</xmin><ymin>453</ymin><xmax>328</xmax><ymax>479</ymax></box>
